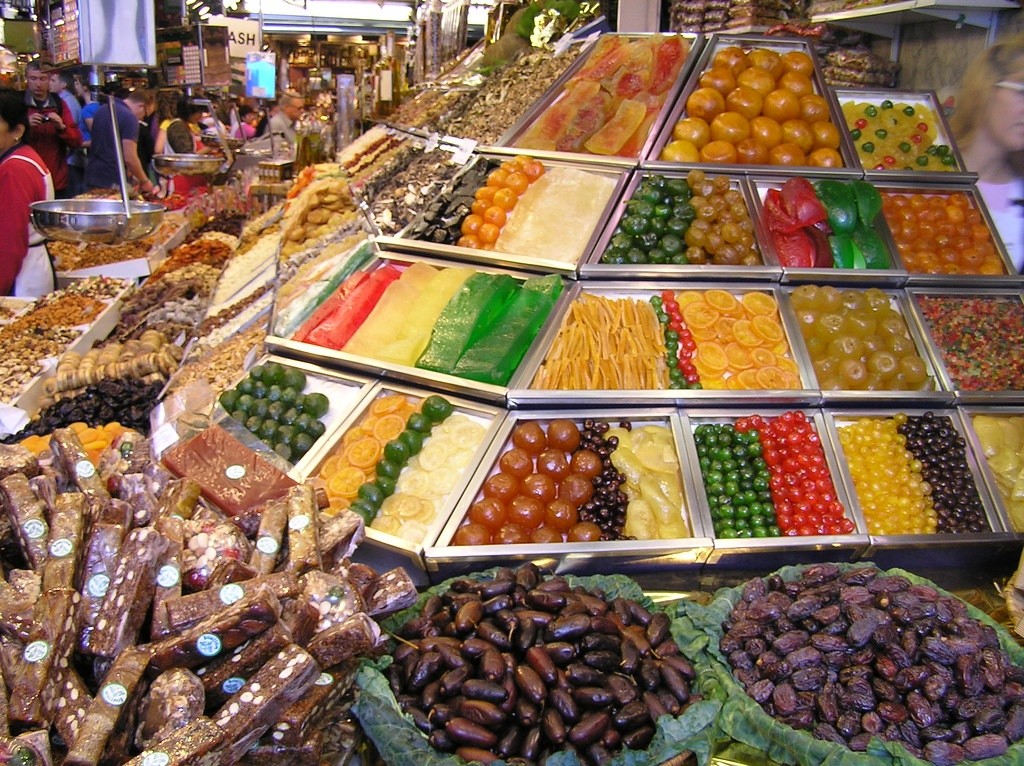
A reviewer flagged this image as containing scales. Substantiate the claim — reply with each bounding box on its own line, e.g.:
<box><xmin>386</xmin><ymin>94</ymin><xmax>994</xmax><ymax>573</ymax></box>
<box><xmin>28</xmin><ymin>0</ymin><xmax>167</xmax><ymax>244</ymax></box>
<box><xmin>150</xmin><ymin>0</ymin><xmax>248</xmax><ymax>179</ymax></box>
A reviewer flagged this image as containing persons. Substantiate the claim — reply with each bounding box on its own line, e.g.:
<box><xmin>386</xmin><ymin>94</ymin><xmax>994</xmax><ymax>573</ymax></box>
<box><xmin>0</xmin><ymin>60</ymin><xmax>305</xmax><ymax>300</ymax></box>
<box><xmin>946</xmin><ymin>33</ymin><xmax>1024</xmax><ymax>275</ymax></box>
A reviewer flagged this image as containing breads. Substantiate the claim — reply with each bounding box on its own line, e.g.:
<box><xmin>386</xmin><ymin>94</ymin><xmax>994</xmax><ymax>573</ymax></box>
<box><xmin>0</xmin><ymin>423</ymin><xmax>422</xmax><ymax>766</ymax></box>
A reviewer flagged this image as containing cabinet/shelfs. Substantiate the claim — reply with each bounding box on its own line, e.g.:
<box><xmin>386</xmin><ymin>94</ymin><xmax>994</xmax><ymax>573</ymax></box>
<box><xmin>33</xmin><ymin>0</ymin><xmax>157</xmax><ymax>72</ymax></box>
<box><xmin>263</xmin><ymin>34</ymin><xmax>380</xmax><ymax>110</ymax></box>
<box><xmin>248</xmin><ymin>83</ymin><xmax>424</xmax><ymax>221</ymax></box>
<box><xmin>1</xmin><ymin>0</ymin><xmax>1024</xmax><ymax>765</ymax></box>
<box><xmin>149</xmin><ymin>26</ymin><xmax>232</xmax><ymax>88</ymax></box>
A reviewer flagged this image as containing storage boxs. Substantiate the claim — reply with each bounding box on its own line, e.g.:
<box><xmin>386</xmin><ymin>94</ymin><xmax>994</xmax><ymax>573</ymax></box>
<box><xmin>33</xmin><ymin>0</ymin><xmax>396</xmax><ymax>221</ymax></box>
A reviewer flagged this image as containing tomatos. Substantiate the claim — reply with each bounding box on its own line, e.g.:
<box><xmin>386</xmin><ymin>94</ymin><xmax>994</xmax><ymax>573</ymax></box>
<box><xmin>661</xmin><ymin>46</ymin><xmax>844</xmax><ymax>170</ymax></box>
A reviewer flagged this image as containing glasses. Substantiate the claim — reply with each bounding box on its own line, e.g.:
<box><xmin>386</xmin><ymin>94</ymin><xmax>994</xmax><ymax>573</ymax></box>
<box><xmin>290</xmin><ymin>104</ymin><xmax>303</xmax><ymax>110</ymax></box>
<box><xmin>143</xmin><ymin>106</ymin><xmax>149</xmax><ymax>120</ymax></box>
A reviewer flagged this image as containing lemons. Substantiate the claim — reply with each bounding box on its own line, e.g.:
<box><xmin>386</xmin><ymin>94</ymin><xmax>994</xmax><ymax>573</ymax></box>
<box><xmin>311</xmin><ymin>395</ymin><xmax>442</xmax><ymax>514</ymax></box>
<box><xmin>677</xmin><ymin>290</ymin><xmax>802</xmax><ymax>389</ymax></box>
